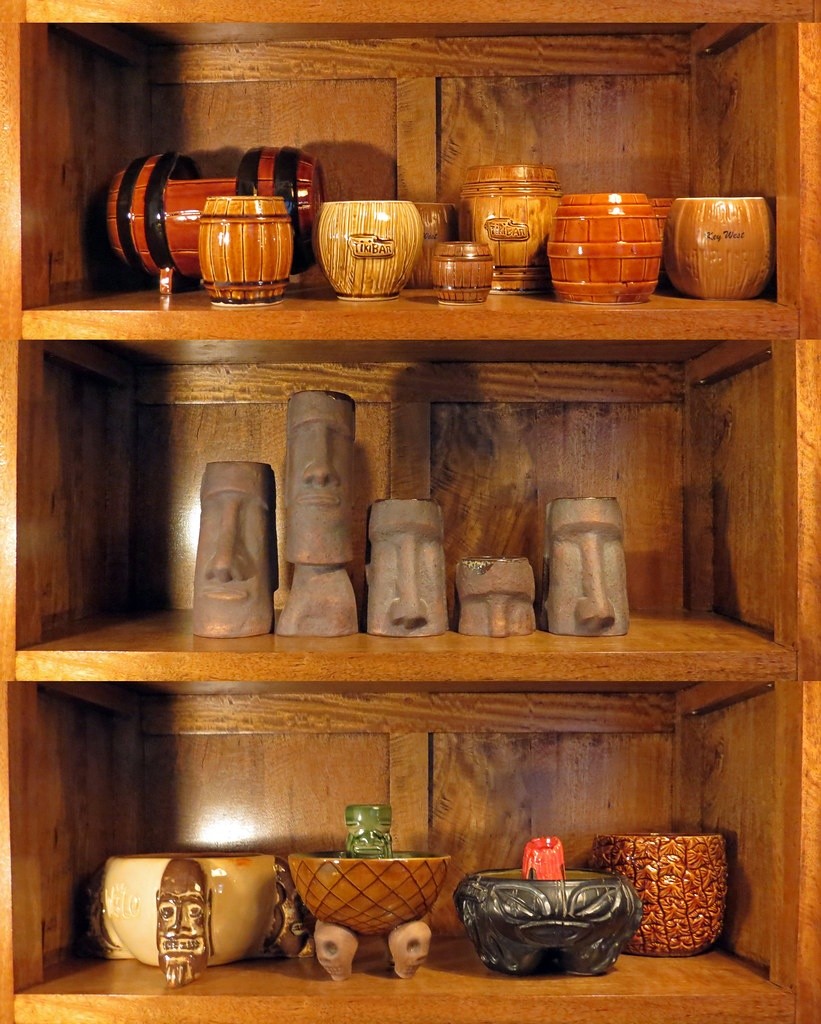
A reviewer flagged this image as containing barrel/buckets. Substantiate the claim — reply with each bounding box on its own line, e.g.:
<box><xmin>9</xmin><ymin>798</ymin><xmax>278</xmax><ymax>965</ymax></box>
<box><xmin>433</xmin><ymin>241</ymin><xmax>494</xmax><ymax>305</ymax></box>
<box><xmin>458</xmin><ymin>165</ymin><xmax>563</xmax><ymax>294</ymax></box>
<box><xmin>649</xmin><ymin>197</ymin><xmax>678</xmax><ymax>273</ymax></box>
<box><xmin>547</xmin><ymin>193</ymin><xmax>663</xmax><ymax>305</ymax></box>
<box><xmin>106</xmin><ymin>151</ymin><xmax>327</xmax><ymax>276</ymax></box>
<box><xmin>198</xmin><ymin>195</ymin><xmax>293</xmax><ymax>307</ymax></box>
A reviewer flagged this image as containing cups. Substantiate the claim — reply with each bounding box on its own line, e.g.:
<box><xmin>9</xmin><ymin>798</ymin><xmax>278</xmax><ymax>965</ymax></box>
<box><xmin>311</xmin><ymin>200</ymin><xmax>423</xmax><ymax>301</ymax></box>
<box><xmin>663</xmin><ymin>197</ymin><xmax>777</xmax><ymax>301</ymax></box>
<box><xmin>401</xmin><ymin>203</ymin><xmax>453</xmax><ymax>286</ymax></box>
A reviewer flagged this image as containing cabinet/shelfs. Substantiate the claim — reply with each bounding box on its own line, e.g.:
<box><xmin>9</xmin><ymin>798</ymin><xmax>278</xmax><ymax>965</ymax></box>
<box><xmin>0</xmin><ymin>0</ymin><xmax>821</xmax><ymax>1024</ymax></box>
